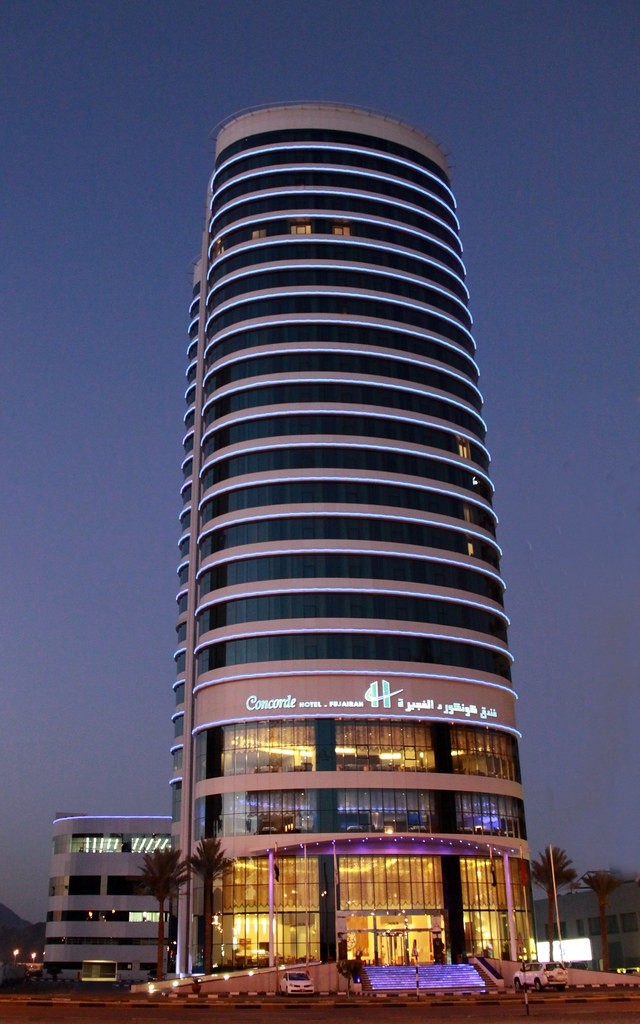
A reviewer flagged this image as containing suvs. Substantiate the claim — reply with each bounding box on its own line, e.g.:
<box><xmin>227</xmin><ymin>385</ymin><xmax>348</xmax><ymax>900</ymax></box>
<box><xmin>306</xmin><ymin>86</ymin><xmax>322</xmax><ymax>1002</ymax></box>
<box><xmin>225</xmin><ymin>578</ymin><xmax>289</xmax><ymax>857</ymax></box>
<box><xmin>513</xmin><ymin>961</ymin><xmax>569</xmax><ymax>992</ymax></box>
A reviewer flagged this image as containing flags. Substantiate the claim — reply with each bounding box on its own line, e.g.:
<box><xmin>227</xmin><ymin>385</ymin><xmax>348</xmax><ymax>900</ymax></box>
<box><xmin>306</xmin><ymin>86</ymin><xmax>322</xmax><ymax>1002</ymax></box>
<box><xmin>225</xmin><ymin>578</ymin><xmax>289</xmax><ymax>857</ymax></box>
<box><xmin>490</xmin><ymin>858</ymin><xmax>497</xmax><ymax>887</ymax></box>
<box><xmin>520</xmin><ymin>855</ymin><xmax>526</xmax><ymax>886</ymax></box>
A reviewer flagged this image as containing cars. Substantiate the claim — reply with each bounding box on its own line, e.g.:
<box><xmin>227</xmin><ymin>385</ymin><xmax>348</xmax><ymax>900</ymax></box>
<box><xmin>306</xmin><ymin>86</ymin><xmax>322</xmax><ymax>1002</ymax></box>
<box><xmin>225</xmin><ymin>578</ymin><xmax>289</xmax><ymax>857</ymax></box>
<box><xmin>279</xmin><ymin>970</ymin><xmax>315</xmax><ymax>997</ymax></box>
<box><xmin>569</xmin><ymin>961</ymin><xmax>589</xmax><ymax>971</ymax></box>
